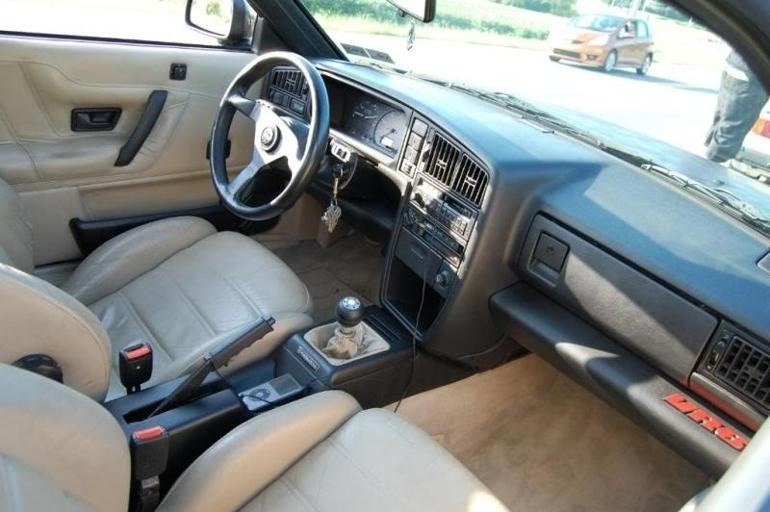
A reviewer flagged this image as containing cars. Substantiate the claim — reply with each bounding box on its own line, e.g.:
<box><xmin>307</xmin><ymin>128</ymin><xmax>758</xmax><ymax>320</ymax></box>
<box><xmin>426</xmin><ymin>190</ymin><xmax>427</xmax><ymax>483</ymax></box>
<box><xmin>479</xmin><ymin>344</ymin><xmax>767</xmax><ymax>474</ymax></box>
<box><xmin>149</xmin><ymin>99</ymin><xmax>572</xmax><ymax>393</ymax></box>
<box><xmin>728</xmin><ymin>97</ymin><xmax>770</xmax><ymax>185</ymax></box>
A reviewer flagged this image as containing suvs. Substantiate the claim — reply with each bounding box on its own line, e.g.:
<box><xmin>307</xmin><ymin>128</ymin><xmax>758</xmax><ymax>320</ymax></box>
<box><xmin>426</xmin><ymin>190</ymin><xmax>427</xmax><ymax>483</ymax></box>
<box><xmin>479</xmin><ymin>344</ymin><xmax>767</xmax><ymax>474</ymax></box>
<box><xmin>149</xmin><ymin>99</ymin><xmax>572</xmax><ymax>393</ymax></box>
<box><xmin>548</xmin><ymin>13</ymin><xmax>657</xmax><ymax>78</ymax></box>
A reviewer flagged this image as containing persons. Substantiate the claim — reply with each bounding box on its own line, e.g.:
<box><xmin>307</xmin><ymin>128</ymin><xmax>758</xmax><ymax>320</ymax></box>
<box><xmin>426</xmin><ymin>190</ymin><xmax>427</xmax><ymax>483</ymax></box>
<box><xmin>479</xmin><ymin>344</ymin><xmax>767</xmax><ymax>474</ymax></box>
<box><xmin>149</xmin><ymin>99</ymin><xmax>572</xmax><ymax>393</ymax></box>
<box><xmin>702</xmin><ymin>45</ymin><xmax>770</xmax><ymax>164</ymax></box>
